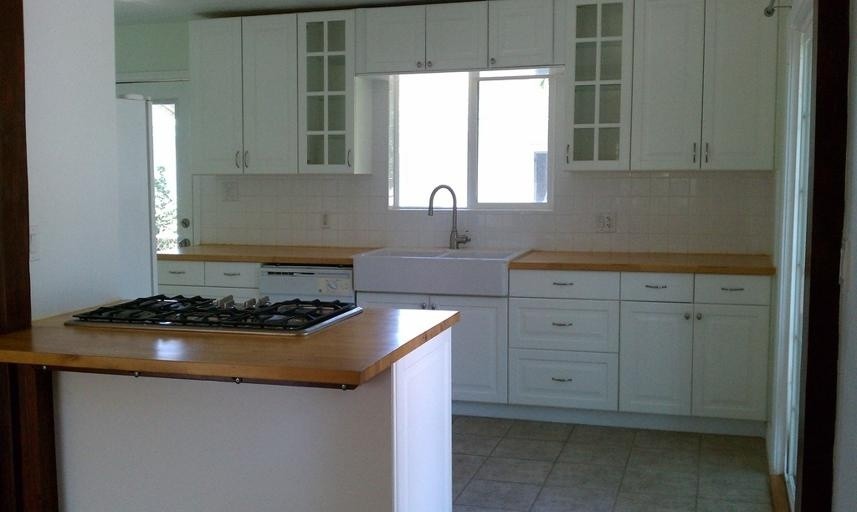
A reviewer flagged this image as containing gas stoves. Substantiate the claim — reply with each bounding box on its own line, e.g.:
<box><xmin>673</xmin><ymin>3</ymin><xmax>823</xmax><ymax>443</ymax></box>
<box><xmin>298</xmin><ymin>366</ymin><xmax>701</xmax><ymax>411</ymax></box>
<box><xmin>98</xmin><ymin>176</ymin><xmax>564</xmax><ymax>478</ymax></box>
<box><xmin>65</xmin><ymin>294</ymin><xmax>364</xmax><ymax>338</ymax></box>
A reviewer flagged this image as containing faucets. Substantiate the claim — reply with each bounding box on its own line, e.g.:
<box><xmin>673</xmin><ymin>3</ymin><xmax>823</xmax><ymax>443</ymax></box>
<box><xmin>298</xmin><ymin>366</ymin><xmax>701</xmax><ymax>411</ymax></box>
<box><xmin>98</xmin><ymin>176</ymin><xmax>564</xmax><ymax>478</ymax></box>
<box><xmin>428</xmin><ymin>184</ymin><xmax>457</xmax><ymax>231</ymax></box>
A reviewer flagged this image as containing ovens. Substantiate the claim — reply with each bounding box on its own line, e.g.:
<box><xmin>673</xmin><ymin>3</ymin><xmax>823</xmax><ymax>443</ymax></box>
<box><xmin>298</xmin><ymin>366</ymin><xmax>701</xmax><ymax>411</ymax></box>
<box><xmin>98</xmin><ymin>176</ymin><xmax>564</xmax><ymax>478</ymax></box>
<box><xmin>258</xmin><ymin>264</ymin><xmax>356</xmax><ymax>305</ymax></box>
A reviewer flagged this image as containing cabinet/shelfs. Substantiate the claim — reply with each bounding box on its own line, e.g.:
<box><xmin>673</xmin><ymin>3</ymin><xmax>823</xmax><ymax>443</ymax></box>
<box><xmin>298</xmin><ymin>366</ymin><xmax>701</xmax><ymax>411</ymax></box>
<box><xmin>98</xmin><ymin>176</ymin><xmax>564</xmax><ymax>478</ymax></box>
<box><xmin>507</xmin><ymin>263</ymin><xmax>617</xmax><ymax>430</ymax></box>
<box><xmin>186</xmin><ymin>13</ymin><xmax>297</xmax><ymax>177</ymax></box>
<box><xmin>559</xmin><ymin>1</ymin><xmax>632</xmax><ymax>175</ymax></box>
<box><xmin>616</xmin><ymin>267</ymin><xmax>776</xmax><ymax>432</ymax></box>
<box><xmin>153</xmin><ymin>256</ymin><xmax>265</xmax><ymax>305</ymax></box>
<box><xmin>632</xmin><ymin>0</ymin><xmax>781</xmax><ymax>177</ymax></box>
<box><xmin>353</xmin><ymin>290</ymin><xmax>509</xmax><ymax>424</ymax></box>
<box><xmin>485</xmin><ymin>1</ymin><xmax>566</xmax><ymax>69</ymax></box>
<box><xmin>352</xmin><ymin>1</ymin><xmax>490</xmax><ymax>78</ymax></box>
<box><xmin>294</xmin><ymin>6</ymin><xmax>376</xmax><ymax>177</ymax></box>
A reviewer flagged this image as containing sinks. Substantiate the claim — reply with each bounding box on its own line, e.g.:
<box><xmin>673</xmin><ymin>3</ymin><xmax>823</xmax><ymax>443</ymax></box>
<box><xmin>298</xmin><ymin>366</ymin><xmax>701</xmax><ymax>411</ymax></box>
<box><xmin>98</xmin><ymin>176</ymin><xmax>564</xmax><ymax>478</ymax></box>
<box><xmin>443</xmin><ymin>248</ymin><xmax>531</xmax><ymax>296</ymax></box>
<box><xmin>352</xmin><ymin>244</ymin><xmax>446</xmax><ymax>294</ymax></box>
